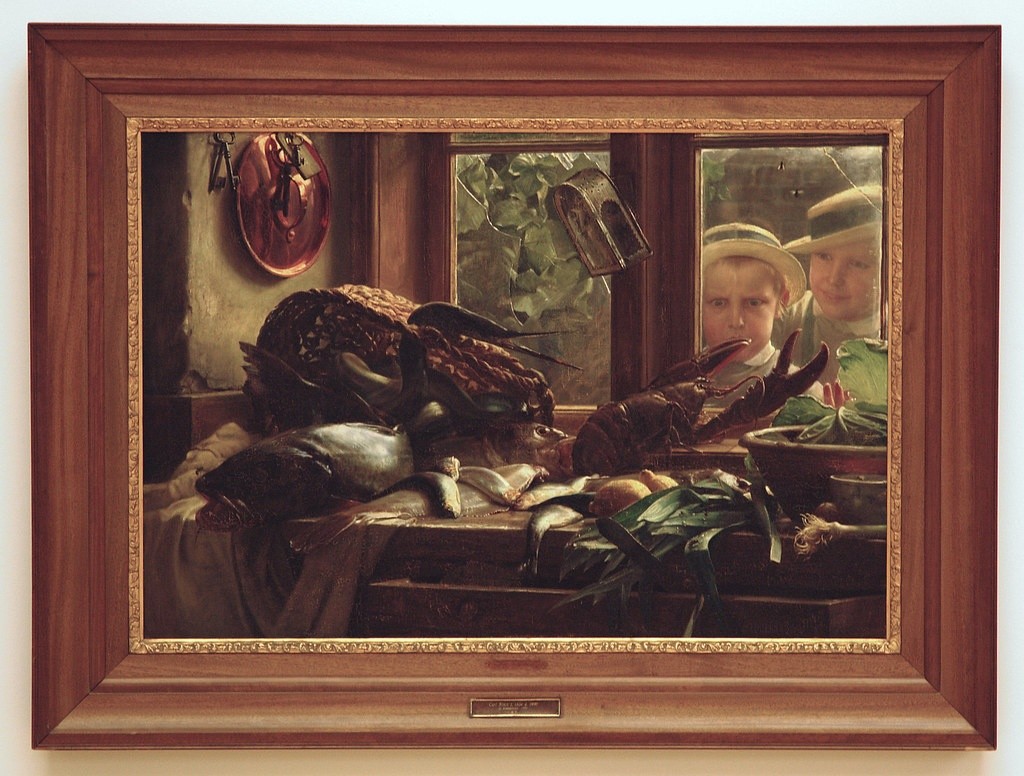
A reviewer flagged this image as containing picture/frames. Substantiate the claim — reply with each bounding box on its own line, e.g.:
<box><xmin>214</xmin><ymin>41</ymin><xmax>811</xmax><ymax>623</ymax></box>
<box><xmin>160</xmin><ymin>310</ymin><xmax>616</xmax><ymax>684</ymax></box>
<box><xmin>27</xmin><ymin>20</ymin><xmax>1001</xmax><ymax>749</ymax></box>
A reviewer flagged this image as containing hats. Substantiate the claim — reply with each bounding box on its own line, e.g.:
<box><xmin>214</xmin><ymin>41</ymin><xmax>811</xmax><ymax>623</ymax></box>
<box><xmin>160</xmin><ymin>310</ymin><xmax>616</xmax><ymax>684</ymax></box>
<box><xmin>783</xmin><ymin>186</ymin><xmax>880</xmax><ymax>254</ymax></box>
<box><xmin>702</xmin><ymin>222</ymin><xmax>807</xmax><ymax>306</ymax></box>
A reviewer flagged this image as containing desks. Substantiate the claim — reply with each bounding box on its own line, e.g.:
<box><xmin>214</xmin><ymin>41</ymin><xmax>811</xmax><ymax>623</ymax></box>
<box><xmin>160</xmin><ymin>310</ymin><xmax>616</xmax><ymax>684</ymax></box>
<box><xmin>145</xmin><ymin>419</ymin><xmax>891</xmax><ymax>636</ymax></box>
